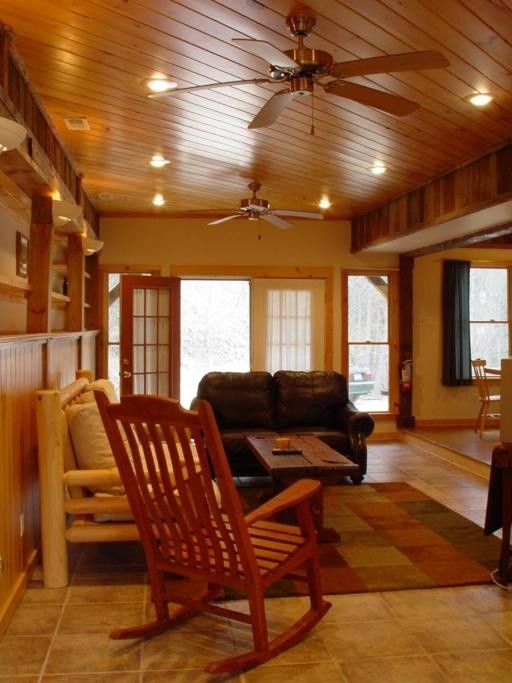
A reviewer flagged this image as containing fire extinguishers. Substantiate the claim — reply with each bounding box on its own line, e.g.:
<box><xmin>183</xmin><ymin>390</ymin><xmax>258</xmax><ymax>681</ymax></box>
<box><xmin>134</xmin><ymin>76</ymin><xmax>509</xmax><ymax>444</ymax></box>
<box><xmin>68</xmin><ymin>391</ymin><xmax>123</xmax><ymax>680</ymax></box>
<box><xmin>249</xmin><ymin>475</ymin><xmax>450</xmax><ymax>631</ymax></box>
<box><xmin>399</xmin><ymin>359</ymin><xmax>413</xmax><ymax>393</ymax></box>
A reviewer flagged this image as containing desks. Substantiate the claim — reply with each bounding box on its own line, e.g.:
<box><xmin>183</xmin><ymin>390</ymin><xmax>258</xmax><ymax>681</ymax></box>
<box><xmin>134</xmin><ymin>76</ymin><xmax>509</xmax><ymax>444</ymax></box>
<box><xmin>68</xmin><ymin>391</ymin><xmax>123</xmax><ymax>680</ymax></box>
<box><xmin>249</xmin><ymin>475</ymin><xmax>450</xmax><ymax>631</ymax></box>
<box><xmin>491</xmin><ymin>442</ymin><xmax>512</xmax><ymax>588</ymax></box>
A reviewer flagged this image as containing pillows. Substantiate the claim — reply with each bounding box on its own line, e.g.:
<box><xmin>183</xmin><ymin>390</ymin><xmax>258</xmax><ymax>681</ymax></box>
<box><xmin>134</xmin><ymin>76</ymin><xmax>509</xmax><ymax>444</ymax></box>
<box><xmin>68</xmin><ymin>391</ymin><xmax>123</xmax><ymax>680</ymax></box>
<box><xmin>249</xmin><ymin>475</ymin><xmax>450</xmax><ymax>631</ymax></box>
<box><xmin>67</xmin><ymin>377</ymin><xmax>139</xmax><ymax>493</ymax></box>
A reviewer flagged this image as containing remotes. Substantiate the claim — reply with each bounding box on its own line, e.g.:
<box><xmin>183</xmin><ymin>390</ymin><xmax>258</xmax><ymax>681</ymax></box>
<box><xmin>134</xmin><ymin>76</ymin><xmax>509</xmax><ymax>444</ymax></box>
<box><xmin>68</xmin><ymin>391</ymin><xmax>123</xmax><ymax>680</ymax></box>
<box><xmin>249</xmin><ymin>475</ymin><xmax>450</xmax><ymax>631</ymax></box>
<box><xmin>271</xmin><ymin>449</ymin><xmax>304</xmax><ymax>454</ymax></box>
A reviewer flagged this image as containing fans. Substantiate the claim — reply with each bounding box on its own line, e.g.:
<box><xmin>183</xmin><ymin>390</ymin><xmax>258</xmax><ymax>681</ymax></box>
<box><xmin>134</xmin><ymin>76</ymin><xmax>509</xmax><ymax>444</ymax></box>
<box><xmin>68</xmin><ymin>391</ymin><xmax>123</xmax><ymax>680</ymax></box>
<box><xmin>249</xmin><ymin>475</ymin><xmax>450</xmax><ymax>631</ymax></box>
<box><xmin>147</xmin><ymin>8</ymin><xmax>452</xmax><ymax>135</ymax></box>
<box><xmin>206</xmin><ymin>183</ymin><xmax>326</xmax><ymax>238</ymax></box>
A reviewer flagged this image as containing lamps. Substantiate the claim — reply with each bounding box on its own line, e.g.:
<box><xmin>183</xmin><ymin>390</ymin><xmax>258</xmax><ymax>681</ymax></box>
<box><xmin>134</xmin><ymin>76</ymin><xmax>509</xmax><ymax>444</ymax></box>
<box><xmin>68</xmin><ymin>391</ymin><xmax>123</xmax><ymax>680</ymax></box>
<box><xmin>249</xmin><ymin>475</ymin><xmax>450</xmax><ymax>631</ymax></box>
<box><xmin>51</xmin><ymin>200</ymin><xmax>82</xmax><ymax>228</ymax></box>
<box><xmin>0</xmin><ymin>116</ymin><xmax>28</xmax><ymax>152</ymax></box>
<box><xmin>82</xmin><ymin>238</ymin><xmax>104</xmax><ymax>256</ymax></box>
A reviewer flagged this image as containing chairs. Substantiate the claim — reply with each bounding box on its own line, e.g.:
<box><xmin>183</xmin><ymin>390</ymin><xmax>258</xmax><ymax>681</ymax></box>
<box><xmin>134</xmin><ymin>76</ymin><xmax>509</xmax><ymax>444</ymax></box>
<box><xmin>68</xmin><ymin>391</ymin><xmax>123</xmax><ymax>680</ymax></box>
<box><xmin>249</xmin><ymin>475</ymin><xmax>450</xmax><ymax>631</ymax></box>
<box><xmin>469</xmin><ymin>358</ymin><xmax>501</xmax><ymax>440</ymax></box>
<box><xmin>91</xmin><ymin>388</ymin><xmax>332</xmax><ymax>677</ymax></box>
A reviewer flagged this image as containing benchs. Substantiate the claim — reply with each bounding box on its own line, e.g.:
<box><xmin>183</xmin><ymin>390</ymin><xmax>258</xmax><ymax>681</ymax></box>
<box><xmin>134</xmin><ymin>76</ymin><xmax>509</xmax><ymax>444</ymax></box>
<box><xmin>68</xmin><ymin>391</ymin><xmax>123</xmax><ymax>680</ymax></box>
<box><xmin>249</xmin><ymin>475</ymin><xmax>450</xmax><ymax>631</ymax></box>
<box><xmin>35</xmin><ymin>370</ymin><xmax>222</xmax><ymax>590</ymax></box>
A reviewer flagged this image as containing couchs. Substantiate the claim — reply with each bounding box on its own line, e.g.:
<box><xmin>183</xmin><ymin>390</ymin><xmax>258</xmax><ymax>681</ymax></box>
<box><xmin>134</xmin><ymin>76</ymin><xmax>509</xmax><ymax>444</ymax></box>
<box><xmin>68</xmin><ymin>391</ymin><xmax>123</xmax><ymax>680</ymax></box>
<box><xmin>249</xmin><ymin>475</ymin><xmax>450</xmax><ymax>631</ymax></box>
<box><xmin>189</xmin><ymin>370</ymin><xmax>374</xmax><ymax>484</ymax></box>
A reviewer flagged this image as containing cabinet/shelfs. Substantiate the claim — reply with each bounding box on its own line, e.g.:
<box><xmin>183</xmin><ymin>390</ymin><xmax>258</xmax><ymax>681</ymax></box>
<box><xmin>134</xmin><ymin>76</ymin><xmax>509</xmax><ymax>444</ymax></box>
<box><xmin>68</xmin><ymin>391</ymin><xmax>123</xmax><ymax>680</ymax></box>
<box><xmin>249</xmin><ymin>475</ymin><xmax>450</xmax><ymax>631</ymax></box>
<box><xmin>0</xmin><ymin>146</ymin><xmax>97</xmax><ymax>335</ymax></box>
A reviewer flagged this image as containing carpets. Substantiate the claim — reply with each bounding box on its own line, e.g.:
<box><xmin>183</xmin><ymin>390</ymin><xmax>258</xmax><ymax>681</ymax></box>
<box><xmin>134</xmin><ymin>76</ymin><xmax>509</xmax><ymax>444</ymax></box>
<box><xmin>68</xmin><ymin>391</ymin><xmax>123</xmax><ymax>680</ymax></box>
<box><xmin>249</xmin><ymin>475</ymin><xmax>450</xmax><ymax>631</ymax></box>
<box><xmin>149</xmin><ymin>480</ymin><xmax>512</xmax><ymax>631</ymax></box>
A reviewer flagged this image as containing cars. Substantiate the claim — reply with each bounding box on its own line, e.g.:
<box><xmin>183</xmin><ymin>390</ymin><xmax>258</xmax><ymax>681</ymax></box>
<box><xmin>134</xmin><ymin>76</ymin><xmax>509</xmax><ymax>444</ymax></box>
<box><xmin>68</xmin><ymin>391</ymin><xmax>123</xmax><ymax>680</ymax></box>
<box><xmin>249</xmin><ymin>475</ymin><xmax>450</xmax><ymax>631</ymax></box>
<box><xmin>348</xmin><ymin>349</ymin><xmax>374</xmax><ymax>401</ymax></box>
